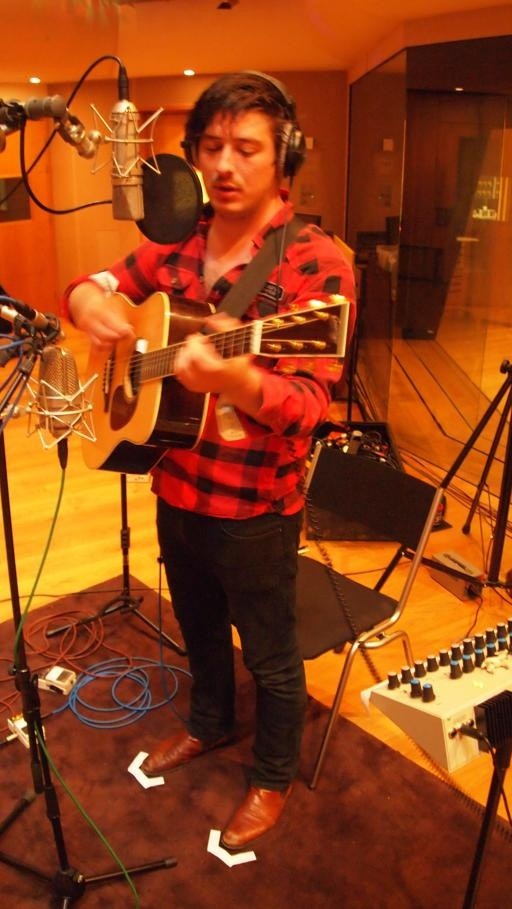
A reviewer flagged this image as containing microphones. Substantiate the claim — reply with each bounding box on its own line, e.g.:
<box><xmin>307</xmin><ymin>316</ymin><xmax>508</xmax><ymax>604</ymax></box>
<box><xmin>38</xmin><ymin>346</ymin><xmax>83</xmax><ymax>471</ymax></box>
<box><xmin>13</xmin><ymin>300</ymin><xmax>65</xmax><ymax>343</ymax></box>
<box><xmin>110</xmin><ymin>67</ymin><xmax>146</xmax><ymax>221</ymax></box>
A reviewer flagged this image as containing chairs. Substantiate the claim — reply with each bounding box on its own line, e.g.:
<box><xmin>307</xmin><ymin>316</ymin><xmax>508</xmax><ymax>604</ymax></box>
<box><xmin>227</xmin><ymin>441</ymin><xmax>442</xmax><ymax>791</ymax></box>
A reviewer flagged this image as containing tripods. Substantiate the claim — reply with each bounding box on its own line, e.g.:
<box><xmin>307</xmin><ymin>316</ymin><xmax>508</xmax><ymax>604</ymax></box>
<box><xmin>374</xmin><ymin>360</ymin><xmax>512</xmax><ymax>594</ymax></box>
<box><xmin>45</xmin><ymin>472</ymin><xmax>187</xmax><ymax>657</ymax></box>
<box><xmin>0</xmin><ymin>435</ymin><xmax>178</xmax><ymax>909</ymax></box>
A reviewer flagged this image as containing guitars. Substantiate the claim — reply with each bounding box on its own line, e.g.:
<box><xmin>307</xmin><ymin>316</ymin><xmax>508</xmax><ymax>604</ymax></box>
<box><xmin>78</xmin><ymin>290</ymin><xmax>351</xmax><ymax>475</ymax></box>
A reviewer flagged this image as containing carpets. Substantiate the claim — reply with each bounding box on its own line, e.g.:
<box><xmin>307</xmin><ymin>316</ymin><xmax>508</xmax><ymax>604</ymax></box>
<box><xmin>1</xmin><ymin>574</ymin><xmax>512</xmax><ymax>908</ymax></box>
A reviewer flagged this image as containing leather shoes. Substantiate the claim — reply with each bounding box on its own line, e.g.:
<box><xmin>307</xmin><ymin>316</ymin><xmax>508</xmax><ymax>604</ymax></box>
<box><xmin>140</xmin><ymin>727</ymin><xmax>236</xmax><ymax>776</ymax></box>
<box><xmin>221</xmin><ymin>781</ymin><xmax>293</xmax><ymax>850</ymax></box>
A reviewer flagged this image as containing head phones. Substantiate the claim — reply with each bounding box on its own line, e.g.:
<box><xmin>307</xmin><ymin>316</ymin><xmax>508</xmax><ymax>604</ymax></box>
<box><xmin>184</xmin><ymin>69</ymin><xmax>306</xmax><ymax>179</ymax></box>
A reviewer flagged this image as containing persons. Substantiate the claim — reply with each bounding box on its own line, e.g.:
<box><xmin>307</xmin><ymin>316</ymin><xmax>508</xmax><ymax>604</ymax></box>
<box><xmin>60</xmin><ymin>70</ymin><xmax>358</xmax><ymax>852</ymax></box>
